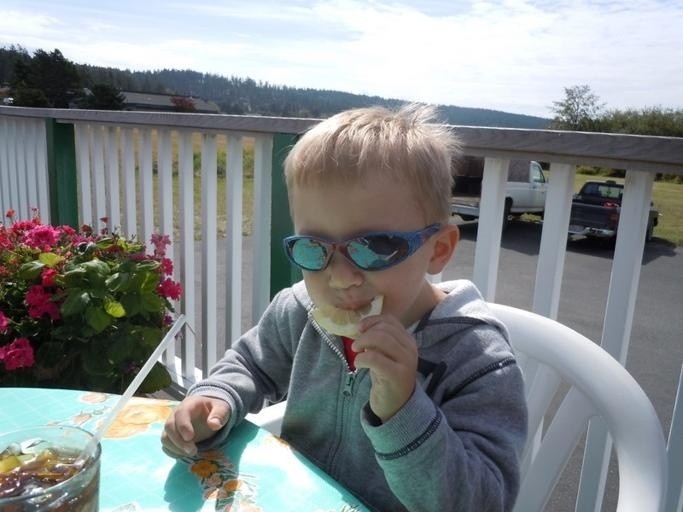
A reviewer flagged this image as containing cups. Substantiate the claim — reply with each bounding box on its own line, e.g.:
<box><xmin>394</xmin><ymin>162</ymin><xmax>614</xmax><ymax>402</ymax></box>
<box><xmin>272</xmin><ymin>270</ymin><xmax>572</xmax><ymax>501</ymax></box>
<box><xmin>0</xmin><ymin>423</ymin><xmax>103</xmax><ymax>512</ymax></box>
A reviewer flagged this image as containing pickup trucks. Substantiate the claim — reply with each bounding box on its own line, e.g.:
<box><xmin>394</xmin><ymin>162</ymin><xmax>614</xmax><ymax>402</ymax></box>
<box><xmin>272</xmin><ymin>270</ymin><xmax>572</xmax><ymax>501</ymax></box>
<box><xmin>569</xmin><ymin>181</ymin><xmax>660</xmax><ymax>244</ymax></box>
<box><xmin>449</xmin><ymin>153</ymin><xmax>548</xmax><ymax>226</ymax></box>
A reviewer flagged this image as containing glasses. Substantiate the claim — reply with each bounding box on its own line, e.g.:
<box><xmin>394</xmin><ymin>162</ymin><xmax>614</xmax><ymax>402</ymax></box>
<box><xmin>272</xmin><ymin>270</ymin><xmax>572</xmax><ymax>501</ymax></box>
<box><xmin>282</xmin><ymin>223</ymin><xmax>442</xmax><ymax>272</ymax></box>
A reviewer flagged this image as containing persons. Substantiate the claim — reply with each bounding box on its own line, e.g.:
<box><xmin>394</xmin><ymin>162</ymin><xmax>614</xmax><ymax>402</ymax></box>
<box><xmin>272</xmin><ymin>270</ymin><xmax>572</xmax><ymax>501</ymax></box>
<box><xmin>161</xmin><ymin>102</ymin><xmax>530</xmax><ymax>512</ymax></box>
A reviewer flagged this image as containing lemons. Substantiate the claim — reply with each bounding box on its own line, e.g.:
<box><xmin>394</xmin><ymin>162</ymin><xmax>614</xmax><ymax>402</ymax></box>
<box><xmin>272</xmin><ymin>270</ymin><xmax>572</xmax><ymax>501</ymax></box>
<box><xmin>312</xmin><ymin>296</ymin><xmax>385</xmax><ymax>339</ymax></box>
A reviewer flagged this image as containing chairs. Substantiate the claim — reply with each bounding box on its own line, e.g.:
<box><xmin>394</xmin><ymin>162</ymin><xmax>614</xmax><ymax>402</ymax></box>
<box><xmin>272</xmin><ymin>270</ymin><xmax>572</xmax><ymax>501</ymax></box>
<box><xmin>244</xmin><ymin>301</ymin><xmax>668</xmax><ymax>512</ymax></box>
